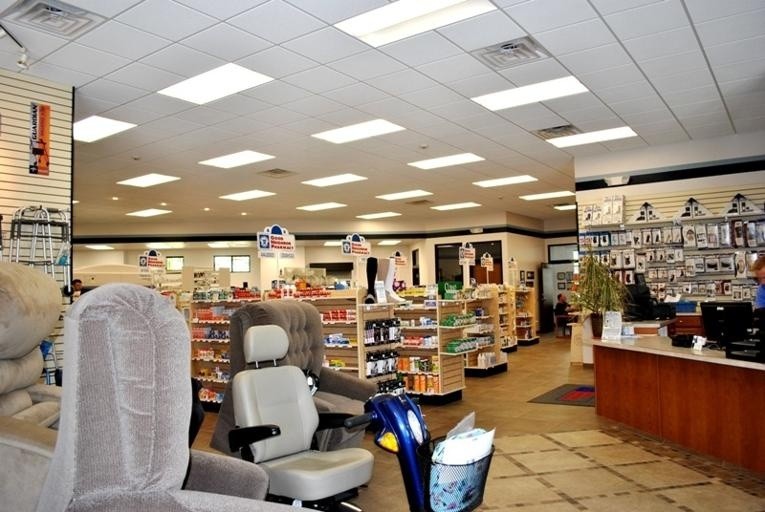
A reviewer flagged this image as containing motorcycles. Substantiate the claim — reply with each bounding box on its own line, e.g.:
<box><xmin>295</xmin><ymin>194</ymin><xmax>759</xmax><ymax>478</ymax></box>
<box><xmin>228</xmin><ymin>324</ymin><xmax>495</xmax><ymax>512</ymax></box>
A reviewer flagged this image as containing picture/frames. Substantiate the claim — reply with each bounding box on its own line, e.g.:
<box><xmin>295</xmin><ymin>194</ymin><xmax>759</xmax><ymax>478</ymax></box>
<box><xmin>557</xmin><ymin>271</ymin><xmax>574</xmax><ymax>290</ymax></box>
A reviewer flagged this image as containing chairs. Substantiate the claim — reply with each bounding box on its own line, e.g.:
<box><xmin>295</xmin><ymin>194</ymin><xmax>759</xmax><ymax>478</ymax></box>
<box><xmin>36</xmin><ymin>281</ymin><xmax>325</xmax><ymax>512</ymax></box>
<box><xmin>226</xmin><ymin>324</ymin><xmax>376</xmax><ymax>512</ymax></box>
<box><xmin>208</xmin><ymin>298</ymin><xmax>379</xmax><ymax>458</ymax></box>
<box><xmin>0</xmin><ymin>261</ymin><xmax>62</xmax><ymax>512</ymax></box>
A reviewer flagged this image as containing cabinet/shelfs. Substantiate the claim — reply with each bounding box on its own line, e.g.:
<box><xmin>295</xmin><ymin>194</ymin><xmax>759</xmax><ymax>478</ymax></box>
<box><xmin>566</xmin><ymin>313</ymin><xmax>583</xmax><ymax>367</ymax></box>
<box><xmin>583</xmin><ymin>210</ymin><xmax>765</xmax><ymax>313</ymax></box>
<box><xmin>635</xmin><ymin>316</ymin><xmax>678</xmax><ymax>336</ymax></box>
<box><xmin>677</xmin><ymin>314</ymin><xmax>704</xmax><ymax>337</ymax></box>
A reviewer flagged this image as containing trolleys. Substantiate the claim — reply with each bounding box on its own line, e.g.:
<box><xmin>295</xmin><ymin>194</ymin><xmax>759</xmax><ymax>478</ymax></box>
<box><xmin>7</xmin><ymin>205</ymin><xmax>72</xmax><ymax>387</ymax></box>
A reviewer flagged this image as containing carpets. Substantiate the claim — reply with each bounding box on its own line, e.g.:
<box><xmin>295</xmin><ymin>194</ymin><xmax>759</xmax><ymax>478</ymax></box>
<box><xmin>527</xmin><ymin>384</ymin><xmax>595</xmax><ymax>407</ymax></box>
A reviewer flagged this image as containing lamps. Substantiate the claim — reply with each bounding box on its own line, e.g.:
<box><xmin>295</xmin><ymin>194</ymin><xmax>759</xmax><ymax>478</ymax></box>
<box><xmin>0</xmin><ymin>20</ymin><xmax>29</xmax><ymax>71</ymax></box>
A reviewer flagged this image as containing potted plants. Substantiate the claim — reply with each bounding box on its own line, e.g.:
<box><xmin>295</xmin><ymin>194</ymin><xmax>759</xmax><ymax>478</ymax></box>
<box><xmin>571</xmin><ymin>244</ymin><xmax>634</xmax><ymax>338</ymax></box>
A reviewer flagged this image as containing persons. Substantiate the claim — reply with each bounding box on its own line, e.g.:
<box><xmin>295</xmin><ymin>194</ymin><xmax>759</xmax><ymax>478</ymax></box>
<box><xmin>70</xmin><ymin>278</ymin><xmax>89</xmax><ymax>301</ymax></box>
<box><xmin>751</xmin><ymin>256</ymin><xmax>765</xmax><ymax>309</ymax></box>
<box><xmin>555</xmin><ymin>294</ymin><xmax>575</xmax><ymax>327</ymax></box>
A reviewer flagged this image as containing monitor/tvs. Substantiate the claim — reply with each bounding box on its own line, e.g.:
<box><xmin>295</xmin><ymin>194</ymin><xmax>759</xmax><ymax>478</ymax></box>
<box><xmin>700</xmin><ymin>301</ymin><xmax>753</xmax><ymax>344</ymax></box>
